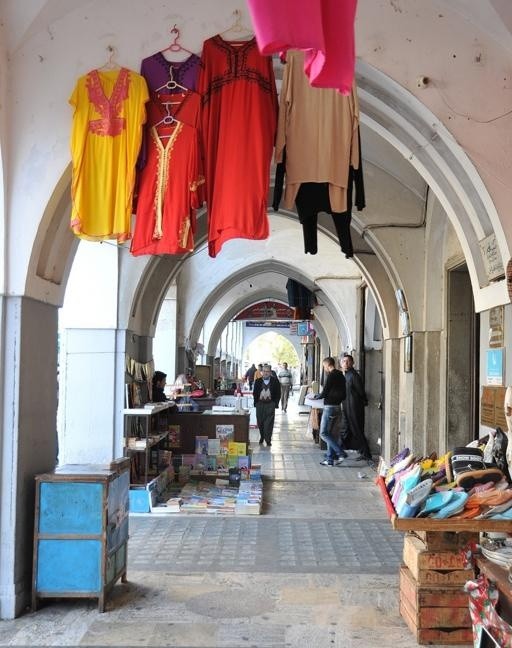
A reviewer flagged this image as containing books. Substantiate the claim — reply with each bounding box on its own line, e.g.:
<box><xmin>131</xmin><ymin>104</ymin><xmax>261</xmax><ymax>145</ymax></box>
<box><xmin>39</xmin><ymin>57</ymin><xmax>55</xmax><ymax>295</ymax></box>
<box><xmin>123</xmin><ymin>380</ymin><xmax>183</xmax><ymax>516</ymax></box>
<box><xmin>184</xmin><ymin>423</ymin><xmax>263</xmax><ymax>516</ymax></box>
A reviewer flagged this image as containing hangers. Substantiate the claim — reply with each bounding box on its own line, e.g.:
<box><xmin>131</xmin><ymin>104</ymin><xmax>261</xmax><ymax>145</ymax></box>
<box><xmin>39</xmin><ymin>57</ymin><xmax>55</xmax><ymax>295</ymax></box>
<box><xmin>94</xmin><ymin>9</ymin><xmax>255</xmax><ymax>139</ymax></box>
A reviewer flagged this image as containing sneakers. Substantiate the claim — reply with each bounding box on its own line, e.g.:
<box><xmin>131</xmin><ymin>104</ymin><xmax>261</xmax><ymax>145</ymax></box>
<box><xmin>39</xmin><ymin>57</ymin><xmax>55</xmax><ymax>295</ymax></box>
<box><xmin>258</xmin><ymin>435</ymin><xmax>275</xmax><ymax>450</ymax></box>
<box><xmin>318</xmin><ymin>453</ymin><xmax>372</xmax><ymax>468</ymax></box>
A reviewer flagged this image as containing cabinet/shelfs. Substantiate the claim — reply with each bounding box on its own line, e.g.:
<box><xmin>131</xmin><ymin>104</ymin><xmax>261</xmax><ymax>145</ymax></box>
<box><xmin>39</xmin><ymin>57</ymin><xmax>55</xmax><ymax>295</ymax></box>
<box><xmin>30</xmin><ymin>461</ymin><xmax>132</xmax><ymax>614</ymax></box>
<box><xmin>124</xmin><ymin>398</ymin><xmax>178</xmax><ymax>513</ymax></box>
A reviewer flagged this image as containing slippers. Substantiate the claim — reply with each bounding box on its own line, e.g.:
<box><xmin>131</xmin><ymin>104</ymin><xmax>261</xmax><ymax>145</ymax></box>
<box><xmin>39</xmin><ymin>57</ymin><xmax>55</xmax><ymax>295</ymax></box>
<box><xmin>461</xmin><ymin>481</ymin><xmax>511</xmax><ymax>519</ymax></box>
<box><xmin>377</xmin><ymin>448</ymin><xmax>468</xmax><ymax>519</ymax></box>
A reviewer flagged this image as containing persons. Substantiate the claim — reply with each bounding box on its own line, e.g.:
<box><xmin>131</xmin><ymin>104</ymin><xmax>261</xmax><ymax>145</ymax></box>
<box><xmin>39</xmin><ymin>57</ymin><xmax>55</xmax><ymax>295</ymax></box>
<box><xmin>242</xmin><ymin>362</ymin><xmax>278</xmax><ymax>391</ymax></box>
<box><xmin>335</xmin><ymin>354</ymin><xmax>374</xmax><ymax>461</ymax></box>
<box><xmin>169</xmin><ymin>388</ymin><xmax>201</xmax><ymax>412</ymax></box>
<box><xmin>307</xmin><ymin>357</ymin><xmax>349</xmax><ymax>468</ymax></box>
<box><xmin>252</xmin><ymin>364</ymin><xmax>281</xmax><ymax>447</ymax></box>
<box><xmin>278</xmin><ymin>362</ymin><xmax>294</xmax><ymax>413</ymax></box>
<box><xmin>152</xmin><ymin>371</ymin><xmax>179</xmax><ymax>417</ymax></box>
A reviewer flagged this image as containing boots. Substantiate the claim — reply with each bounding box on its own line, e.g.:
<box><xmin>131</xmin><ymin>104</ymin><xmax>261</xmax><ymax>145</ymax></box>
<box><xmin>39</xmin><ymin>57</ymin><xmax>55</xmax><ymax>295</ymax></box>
<box><xmin>450</xmin><ymin>445</ymin><xmax>504</xmax><ymax>489</ymax></box>
